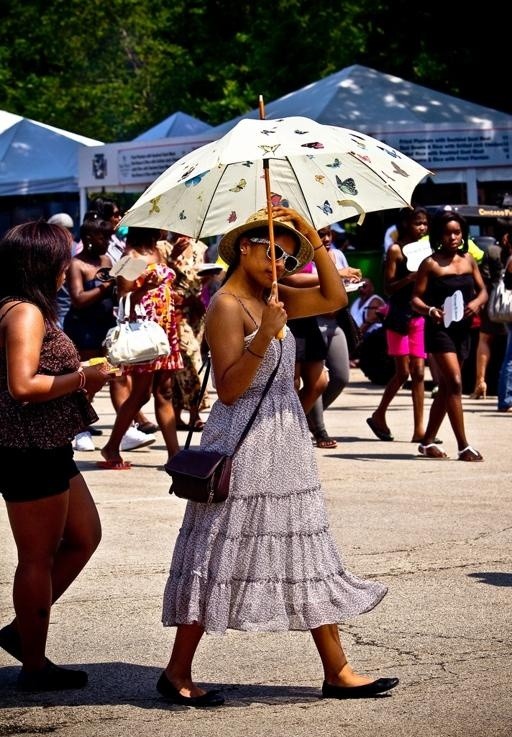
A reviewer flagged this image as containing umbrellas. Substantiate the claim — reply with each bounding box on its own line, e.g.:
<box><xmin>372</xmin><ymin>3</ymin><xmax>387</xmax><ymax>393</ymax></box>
<box><xmin>113</xmin><ymin>94</ymin><xmax>436</xmax><ymax>341</ymax></box>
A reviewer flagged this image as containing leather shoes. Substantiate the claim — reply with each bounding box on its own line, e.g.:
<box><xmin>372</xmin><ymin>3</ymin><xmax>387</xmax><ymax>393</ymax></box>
<box><xmin>322</xmin><ymin>678</ymin><xmax>399</xmax><ymax>698</ymax></box>
<box><xmin>156</xmin><ymin>672</ymin><xmax>225</xmax><ymax>706</ymax></box>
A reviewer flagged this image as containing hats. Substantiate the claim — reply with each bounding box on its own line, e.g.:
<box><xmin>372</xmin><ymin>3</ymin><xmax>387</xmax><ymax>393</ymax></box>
<box><xmin>218</xmin><ymin>208</ymin><xmax>314</xmax><ymax>279</ymax></box>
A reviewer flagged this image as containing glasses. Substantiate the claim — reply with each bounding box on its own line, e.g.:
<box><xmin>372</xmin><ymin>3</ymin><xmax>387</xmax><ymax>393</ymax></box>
<box><xmin>250</xmin><ymin>238</ymin><xmax>302</xmax><ymax>273</ymax></box>
<box><xmin>112</xmin><ymin>211</ymin><xmax>120</xmax><ymax>218</ymax></box>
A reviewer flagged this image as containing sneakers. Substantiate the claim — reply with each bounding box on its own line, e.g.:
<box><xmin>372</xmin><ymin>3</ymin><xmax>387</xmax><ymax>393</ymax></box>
<box><xmin>70</xmin><ymin>430</ymin><xmax>151</xmax><ymax>448</ymax></box>
<box><xmin>0</xmin><ymin>624</ymin><xmax>25</xmax><ymax>664</ymax></box>
<box><xmin>15</xmin><ymin>658</ymin><xmax>87</xmax><ymax>691</ymax></box>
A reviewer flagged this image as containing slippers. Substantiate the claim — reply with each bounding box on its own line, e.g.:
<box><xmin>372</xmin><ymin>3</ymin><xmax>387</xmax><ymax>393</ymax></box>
<box><xmin>97</xmin><ymin>422</ymin><xmax>211</xmax><ymax>470</ymax></box>
<box><xmin>367</xmin><ymin>417</ymin><xmax>485</xmax><ymax>462</ymax></box>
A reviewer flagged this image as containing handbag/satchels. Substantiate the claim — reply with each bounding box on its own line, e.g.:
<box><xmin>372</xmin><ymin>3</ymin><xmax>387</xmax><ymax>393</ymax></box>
<box><xmin>164</xmin><ymin>450</ymin><xmax>232</xmax><ymax>503</ymax></box>
<box><xmin>488</xmin><ymin>278</ymin><xmax>512</xmax><ymax>322</ymax></box>
<box><xmin>101</xmin><ymin>292</ymin><xmax>172</xmax><ymax>365</ymax></box>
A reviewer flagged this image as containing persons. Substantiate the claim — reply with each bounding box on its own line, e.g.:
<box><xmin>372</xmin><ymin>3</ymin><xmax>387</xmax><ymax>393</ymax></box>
<box><xmin>0</xmin><ymin>222</ymin><xmax>118</xmax><ymax>692</ymax></box>
<box><xmin>47</xmin><ymin>197</ymin><xmax>512</xmax><ymax>470</ymax></box>
<box><xmin>154</xmin><ymin>206</ymin><xmax>400</xmax><ymax>707</ymax></box>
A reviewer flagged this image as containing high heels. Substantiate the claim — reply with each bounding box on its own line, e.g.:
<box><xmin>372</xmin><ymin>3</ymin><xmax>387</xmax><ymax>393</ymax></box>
<box><xmin>468</xmin><ymin>381</ymin><xmax>487</xmax><ymax>399</ymax></box>
